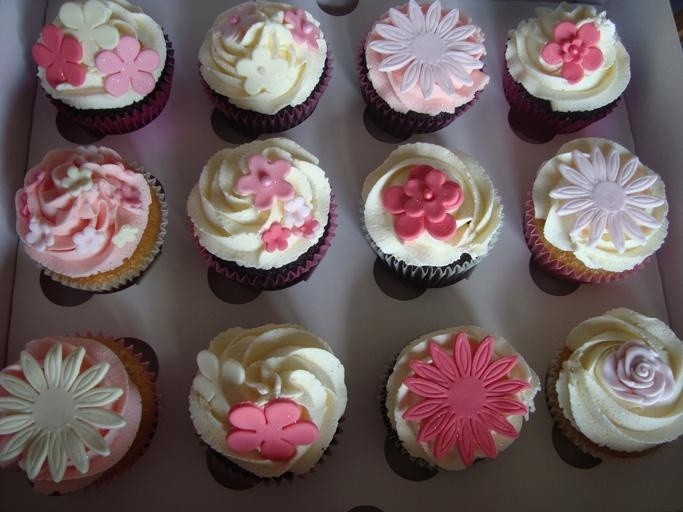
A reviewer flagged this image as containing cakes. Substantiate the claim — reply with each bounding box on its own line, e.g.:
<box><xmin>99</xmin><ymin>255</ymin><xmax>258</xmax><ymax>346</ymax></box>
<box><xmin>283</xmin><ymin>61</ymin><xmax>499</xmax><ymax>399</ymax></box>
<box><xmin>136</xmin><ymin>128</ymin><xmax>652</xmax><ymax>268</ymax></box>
<box><xmin>384</xmin><ymin>325</ymin><xmax>542</xmax><ymax>475</ymax></box>
<box><xmin>186</xmin><ymin>138</ymin><xmax>339</xmax><ymax>292</ymax></box>
<box><xmin>199</xmin><ymin>2</ymin><xmax>332</xmax><ymax>135</ymax></box>
<box><xmin>2</xmin><ymin>334</ymin><xmax>164</xmax><ymax>500</ymax></box>
<box><xmin>357</xmin><ymin>142</ymin><xmax>505</xmax><ymax>284</ymax></box>
<box><xmin>546</xmin><ymin>306</ymin><xmax>682</xmax><ymax>464</ymax></box>
<box><xmin>526</xmin><ymin>137</ymin><xmax>669</xmax><ymax>284</ymax></box>
<box><xmin>31</xmin><ymin>2</ymin><xmax>179</xmax><ymax>137</ymax></box>
<box><xmin>502</xmin><ymin>4</ymin><xmax>634</xmax><ymax>131</ymax></box>
<box><xmin>12</xmin><ymin>144</ymin><xmax>170</xmax><ymax>294</ymax></box>
<box><xmin>355</xmin><ymin>0</ymin><xmax>488</xmax><ymax>135</ymax></box>
<box><xmin>189</xmin><ymin>324</ymin><xmax>350</xmax><ymax>479</ymax></box>
